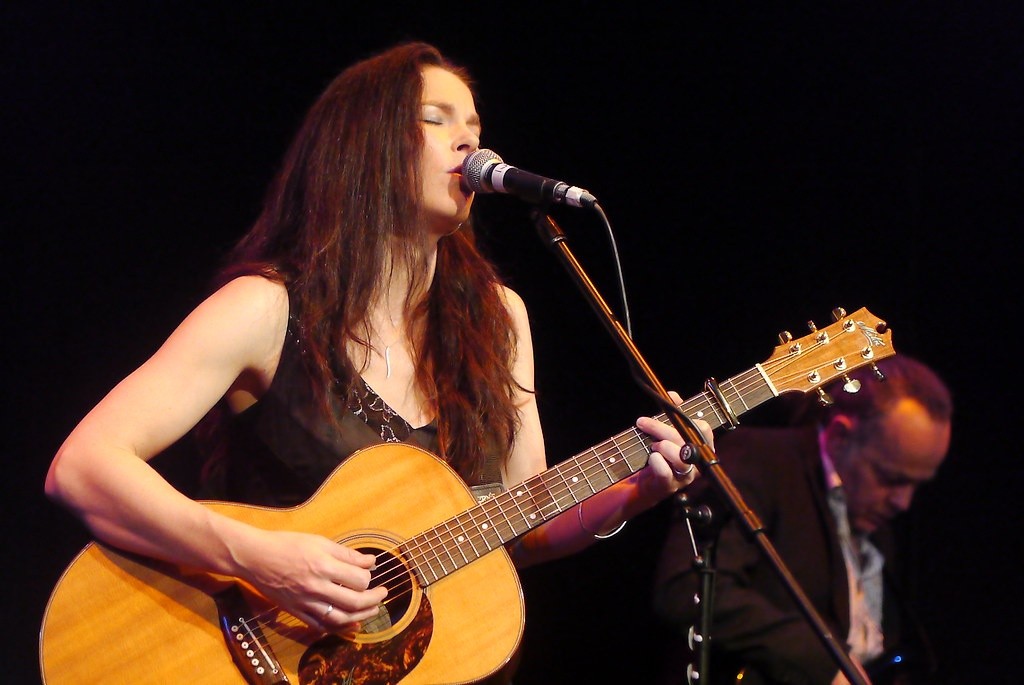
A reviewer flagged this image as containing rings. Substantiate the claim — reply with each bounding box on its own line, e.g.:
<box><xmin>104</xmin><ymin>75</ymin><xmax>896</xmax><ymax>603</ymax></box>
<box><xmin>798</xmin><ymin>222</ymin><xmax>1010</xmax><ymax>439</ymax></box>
<box><xmin>674</xmin><ymin>464</ymin><xmax>695</xmax><ymax>477</ymax></box>
<box><xmin>319</xmin><ymin>603</ymin><xmax>334</xmax><ymax>621</ymax></box>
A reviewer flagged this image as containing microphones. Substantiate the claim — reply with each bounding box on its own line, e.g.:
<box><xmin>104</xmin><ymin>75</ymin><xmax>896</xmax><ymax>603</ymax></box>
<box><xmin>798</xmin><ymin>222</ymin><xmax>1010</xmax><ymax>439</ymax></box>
<box><xmin>462</xmin><ymin>149</ymin><xmax>598</xmax><ymax>207</ymax></box>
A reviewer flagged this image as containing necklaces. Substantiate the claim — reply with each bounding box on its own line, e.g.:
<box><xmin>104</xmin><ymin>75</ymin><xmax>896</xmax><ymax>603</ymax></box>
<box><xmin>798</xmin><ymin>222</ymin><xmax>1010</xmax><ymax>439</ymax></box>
<box><xmin>365</xmin><ymin>315</ymin><xmax>409</xmax><ymax>380</ymax></box>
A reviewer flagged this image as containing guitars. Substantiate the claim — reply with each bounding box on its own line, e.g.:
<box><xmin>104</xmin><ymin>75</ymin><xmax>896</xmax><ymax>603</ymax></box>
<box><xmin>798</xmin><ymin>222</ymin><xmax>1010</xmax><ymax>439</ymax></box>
<box><xmin>37</xmin><ymin>300</ymin><xmax>898</xmax><ymax>685</ymax></box>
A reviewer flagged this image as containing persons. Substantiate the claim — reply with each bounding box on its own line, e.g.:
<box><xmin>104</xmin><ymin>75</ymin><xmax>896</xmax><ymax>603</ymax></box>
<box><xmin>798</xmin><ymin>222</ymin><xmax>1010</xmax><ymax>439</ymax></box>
<box><xmin>644</xmin><ymin>352</ymin><xmax>954</xmax><ymax>685</ymax></box>
<box><xmin>37</xmin><ymin>38</ymin><xmax>718</xmax><ymax>632</ymax></box>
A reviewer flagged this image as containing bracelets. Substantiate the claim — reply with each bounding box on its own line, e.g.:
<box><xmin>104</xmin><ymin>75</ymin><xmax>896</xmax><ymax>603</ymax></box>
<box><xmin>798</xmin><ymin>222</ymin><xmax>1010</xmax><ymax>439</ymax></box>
<box><xmin>578</xmin><ymin>502</ymin><xmax>631</xmax><ymax>541</ymax></box>
